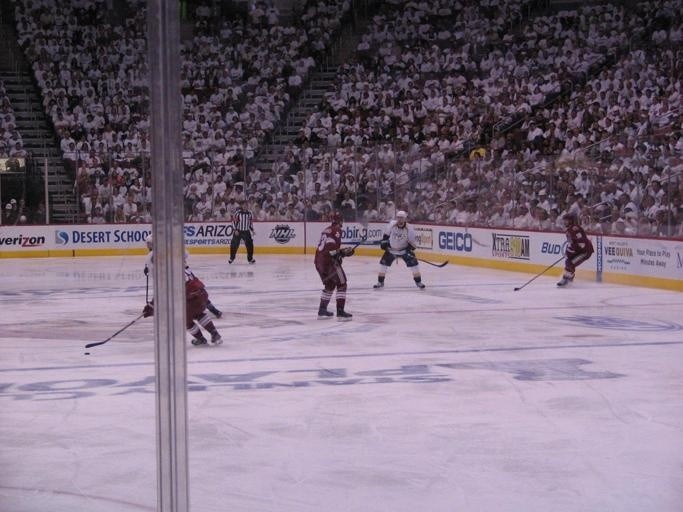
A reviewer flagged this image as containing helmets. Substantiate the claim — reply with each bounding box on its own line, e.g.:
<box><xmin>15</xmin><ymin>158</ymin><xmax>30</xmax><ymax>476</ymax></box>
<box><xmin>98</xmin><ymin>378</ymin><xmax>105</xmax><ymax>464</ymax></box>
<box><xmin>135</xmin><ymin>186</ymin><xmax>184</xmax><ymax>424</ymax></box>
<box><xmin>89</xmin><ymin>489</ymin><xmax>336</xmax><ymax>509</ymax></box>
<box><xmin>329</xmin><ymin>210</ymin><xmax>343</xmax><ymax>225</ymax></box>
<box><xmin>563</xmin><ymin>213</ymin><xmax>573</xmax><ymax>227</ymax></box>
<box><xmin>397</xmin><ymin>211</ymin><xmax>406</xmax><ymax>225</ymax></box>
<box><xmin>147</xmin><ymin>234</ymin><xmax>153</xmax><ymax>242</ymax></box>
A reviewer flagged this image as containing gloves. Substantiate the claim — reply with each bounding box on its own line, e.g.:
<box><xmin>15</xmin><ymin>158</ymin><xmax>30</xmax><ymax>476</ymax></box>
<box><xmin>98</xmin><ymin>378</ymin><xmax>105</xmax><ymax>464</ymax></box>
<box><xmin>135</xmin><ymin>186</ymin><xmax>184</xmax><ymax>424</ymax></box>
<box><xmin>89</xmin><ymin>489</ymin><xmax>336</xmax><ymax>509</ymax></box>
<box><xmin>566</xmin><ymin>244</ymin><xmax>575</xmax><ymax>256</ymax></box>
<box><xmin>143</xmin><ymin>302</ymin><xmax>153</xmax><ymax>317</ymax></box>
<box><xmin>380</xmin><ymin>240</ymin><xmax>389</xmax><ymax>249</ymax></box>
<box><xmin>341</xmin><ymin>247</ymin><xmax>354</xmax><ymax>256</ymax></box>
<box><xmin>407</xmin><ymin>248</ymin><xmax>415</xmax><ymax>259</ymax></box>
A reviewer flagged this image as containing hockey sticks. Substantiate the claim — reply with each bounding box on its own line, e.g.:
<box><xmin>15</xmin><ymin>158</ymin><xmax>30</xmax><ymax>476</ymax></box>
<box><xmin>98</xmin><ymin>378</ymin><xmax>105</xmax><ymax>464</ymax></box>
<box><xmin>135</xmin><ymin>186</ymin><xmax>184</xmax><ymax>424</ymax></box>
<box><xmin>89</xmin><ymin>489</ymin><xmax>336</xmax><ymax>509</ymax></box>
<box><xmin>515</xmin><ymin>257</ymin><xmax>564</xmax><ymax>291</ymax></box>
<box><xmin>85</xmin><ymin>311</ymin><xmax>148</xmax><ymax>356</ymax></box>
<box><xmin>350</xmin><ymin>229</ymin><xmax>367</xmax><ymax>250</ymax></box>
<box><xmin>411</xmin><ymin>256</ymin><xmax>449</xmax><ymax>267</ymax></box>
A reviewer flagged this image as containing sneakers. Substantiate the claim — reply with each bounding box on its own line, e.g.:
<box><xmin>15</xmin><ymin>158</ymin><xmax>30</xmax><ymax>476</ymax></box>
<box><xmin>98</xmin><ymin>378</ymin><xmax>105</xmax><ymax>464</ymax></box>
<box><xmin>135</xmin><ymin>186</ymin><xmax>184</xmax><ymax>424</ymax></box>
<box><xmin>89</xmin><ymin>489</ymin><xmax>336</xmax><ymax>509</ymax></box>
<box><xmin>416</xmin><ymin>281</ymin><xmax>425</xmax><ymax>288</ymax></box>
<box><xmin>374</xmin><ymin>281</ymin><xmax>384</xmax><ymax>288</ymax></box>
<box><xmin>557</xmin><ymin>272</ymin><xmax>574</xmax><ymax>284</ymax></box>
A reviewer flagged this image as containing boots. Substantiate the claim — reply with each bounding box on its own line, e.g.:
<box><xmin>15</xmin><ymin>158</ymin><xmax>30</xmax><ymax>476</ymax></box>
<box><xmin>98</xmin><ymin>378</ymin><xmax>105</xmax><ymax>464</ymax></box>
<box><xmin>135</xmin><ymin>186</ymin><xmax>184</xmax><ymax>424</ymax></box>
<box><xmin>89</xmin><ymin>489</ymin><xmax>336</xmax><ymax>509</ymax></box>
<box><xmin>192</xmin><ymin>336</ymin><xmax>207</xmax><ymax>344</ymax></box>
<box><xmin>214</xmin><ymin>310</ymin><xmax>221</xmax><ymax>318</ymax></box>
<box><xmin>337</xmin><ymin>307</ymin><xmax>352</xmax><ymax>317</ymax></box>
<box><xmin>318</xmin><ymin>304</ymin><xmax>332</xmax><ymax>316</ymax></box>
<box><xmin>211</xmin><ymin>330</ymin><xmax>220</xmax><ymax>342</ymax></box>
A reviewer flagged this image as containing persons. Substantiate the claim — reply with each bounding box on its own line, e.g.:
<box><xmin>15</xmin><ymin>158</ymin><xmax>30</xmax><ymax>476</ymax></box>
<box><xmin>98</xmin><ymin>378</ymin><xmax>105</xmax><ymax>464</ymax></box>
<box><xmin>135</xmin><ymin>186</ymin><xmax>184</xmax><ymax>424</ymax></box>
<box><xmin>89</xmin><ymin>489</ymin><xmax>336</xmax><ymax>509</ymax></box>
<box><xmin>143</xmin><ymin>235</ymin><xmax>221</xmax><ymax>344</ymax></box>
<box><xmin>1</xmin><ymin>1</ymin><xmax>683</xmax><ymax>238</ymax></box>
<box><xmin>141</xmin><ymin>233</ymin><xmax>223</xmax><ymax>320</ymax></box>
<box><xmin>315</xmin><ymin>211</ymin><xmax>353</xmax><ymax>317</ymax></box>
<box><xmin>373</xmin><ymin>210</ymin><xmax>426</xmax><ymax>289</ymax></box>
<box><xmin>228</xmin><ymin>199</ymin><xmax>256</xmax><ymax>263</ymax></box>
<box><xmin>556</xmin><ymin>213</ymin><xmax>594</xmax><ymax>286</ymax></box>
